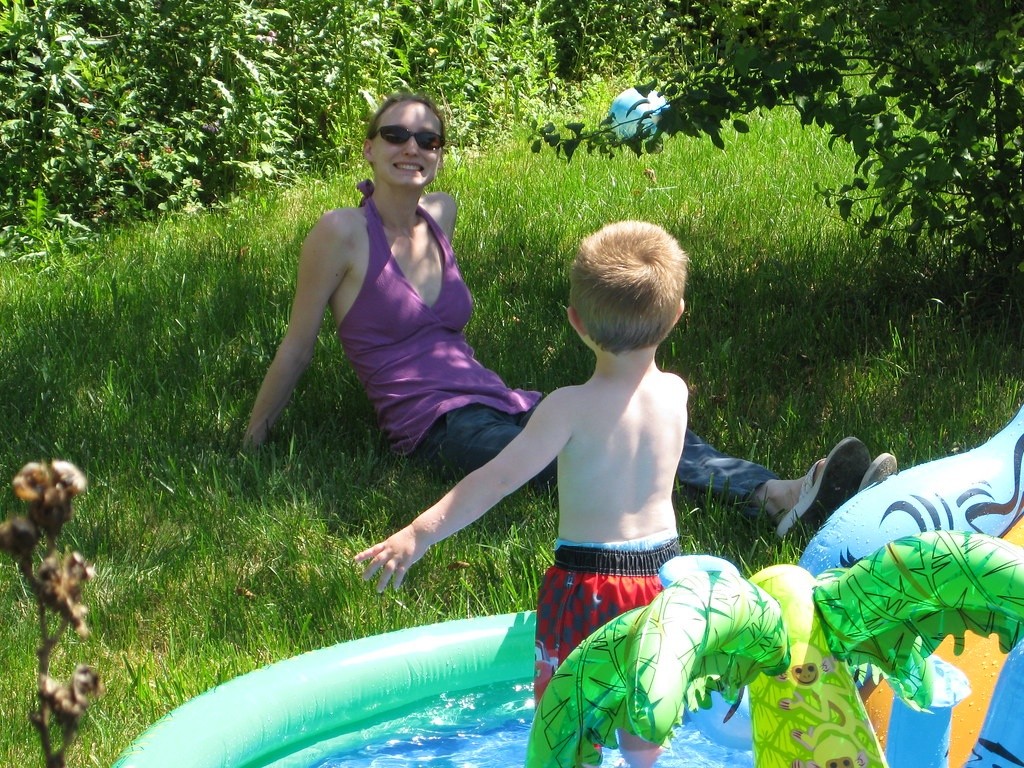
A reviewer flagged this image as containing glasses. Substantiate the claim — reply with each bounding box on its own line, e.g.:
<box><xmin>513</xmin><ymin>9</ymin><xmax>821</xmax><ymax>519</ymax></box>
<box><xmin>370</xmin><ymin>125</ymin><xmax>447</xmax><ymax>150</ymax></box>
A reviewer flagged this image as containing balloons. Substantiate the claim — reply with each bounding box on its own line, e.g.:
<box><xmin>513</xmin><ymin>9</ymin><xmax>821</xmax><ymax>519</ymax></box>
<box><xmin>610</xmin><ymin>88</ymin><xmax>671</xmax><ymax>139</ymax></box>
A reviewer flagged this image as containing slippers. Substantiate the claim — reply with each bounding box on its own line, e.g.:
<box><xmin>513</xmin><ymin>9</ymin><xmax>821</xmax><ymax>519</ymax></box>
<box><xmin>776</xmin><ymin>437</ymin><xmax>872</xmax><ymax>544</ymax></box>
<box><xmin>858</xmin><ymin>453</ymin><xmax>898</xmax><ymax>493</ymax></box>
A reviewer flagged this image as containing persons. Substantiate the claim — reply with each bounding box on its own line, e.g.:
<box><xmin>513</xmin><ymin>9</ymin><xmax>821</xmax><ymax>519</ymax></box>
<box><xmin>241</xmin><ymin>94</ymin><xmax>897</xmax><ymax>542</ymax></box>
<box><xmin>352</xmin><ymin>221</ymin><xmax>689</xmax><ymax>767</ymax></box>
